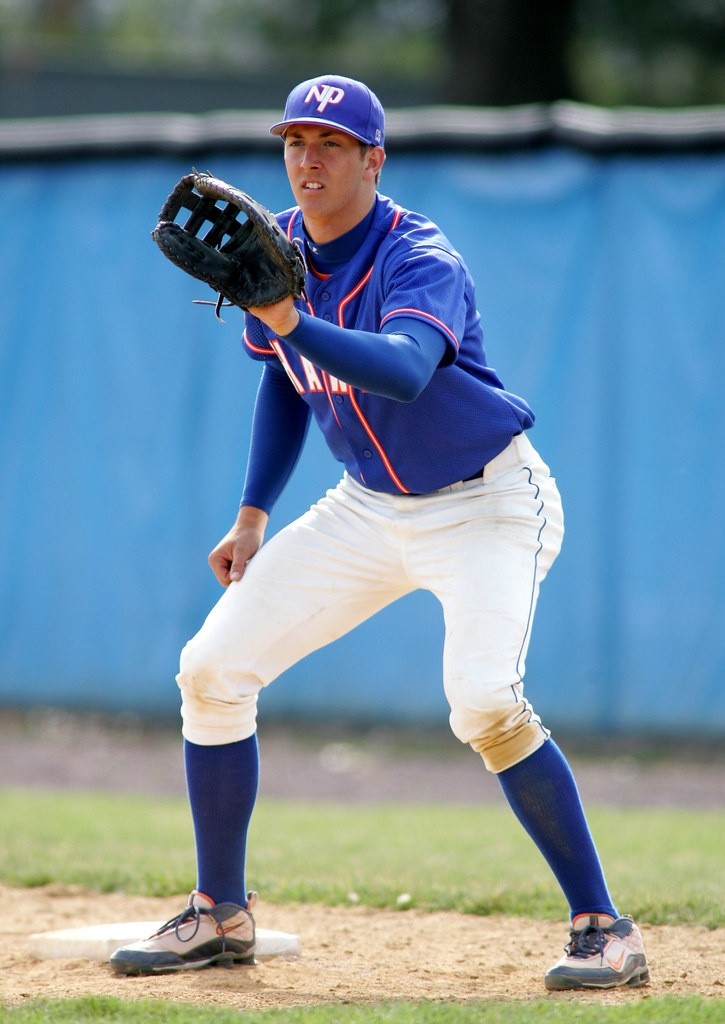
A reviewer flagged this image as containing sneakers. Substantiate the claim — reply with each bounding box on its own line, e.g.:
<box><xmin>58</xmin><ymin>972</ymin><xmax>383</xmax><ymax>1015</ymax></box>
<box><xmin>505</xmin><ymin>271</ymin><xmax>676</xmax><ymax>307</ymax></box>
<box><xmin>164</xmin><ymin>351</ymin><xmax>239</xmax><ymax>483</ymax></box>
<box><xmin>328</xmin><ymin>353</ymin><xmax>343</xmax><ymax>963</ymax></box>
<box><xmin>544</xmin><ymin>911</ymin><xmax>650</xmax><ymax>992</ymax></box>
<box><xmin>110</xmin><ymin>887</ymin><xmax>258</xmax><ymax>976</ymax></box>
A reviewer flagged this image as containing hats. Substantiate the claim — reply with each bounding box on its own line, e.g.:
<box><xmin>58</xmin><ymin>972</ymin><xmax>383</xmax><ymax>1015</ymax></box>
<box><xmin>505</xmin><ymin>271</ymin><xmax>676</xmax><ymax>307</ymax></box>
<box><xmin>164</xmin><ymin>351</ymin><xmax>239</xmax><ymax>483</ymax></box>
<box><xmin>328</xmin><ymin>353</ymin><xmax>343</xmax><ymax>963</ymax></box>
<box><xmin>269</xmin><ymin>74</ymin><xmax>385</xmax><ymax>152</ymax></box>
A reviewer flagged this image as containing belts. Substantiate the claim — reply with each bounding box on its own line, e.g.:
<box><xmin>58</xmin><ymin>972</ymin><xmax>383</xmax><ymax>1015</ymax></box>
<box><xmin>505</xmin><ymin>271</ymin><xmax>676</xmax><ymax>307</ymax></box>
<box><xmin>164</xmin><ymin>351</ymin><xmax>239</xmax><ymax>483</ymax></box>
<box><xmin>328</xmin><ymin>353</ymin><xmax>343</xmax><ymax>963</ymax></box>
<box><xmin>463</xmin><ymin>466</ymin><xmax>485</xmax><ymax>482</ymax></box>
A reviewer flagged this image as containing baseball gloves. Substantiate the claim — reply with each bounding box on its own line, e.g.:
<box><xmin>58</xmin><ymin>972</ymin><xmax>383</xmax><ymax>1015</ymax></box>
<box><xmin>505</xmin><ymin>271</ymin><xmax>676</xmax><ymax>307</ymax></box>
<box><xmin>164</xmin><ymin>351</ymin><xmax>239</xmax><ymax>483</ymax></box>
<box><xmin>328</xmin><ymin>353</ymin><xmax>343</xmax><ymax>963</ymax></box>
<box><xmin>150</xmin><ymin>166</ymin><xmax>309</xmax><ymax>325</ymax></box>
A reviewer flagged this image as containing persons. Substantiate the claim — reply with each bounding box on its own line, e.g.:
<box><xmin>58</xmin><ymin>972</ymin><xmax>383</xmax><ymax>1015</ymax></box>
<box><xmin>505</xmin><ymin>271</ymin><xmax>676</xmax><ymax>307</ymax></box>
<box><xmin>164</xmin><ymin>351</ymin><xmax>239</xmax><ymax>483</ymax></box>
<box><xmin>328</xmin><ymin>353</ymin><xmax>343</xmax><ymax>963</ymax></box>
<box><xmin>111</xmin><ymin>75</ymin><xmax>648</xmax><ymax>992</ymax></box>
